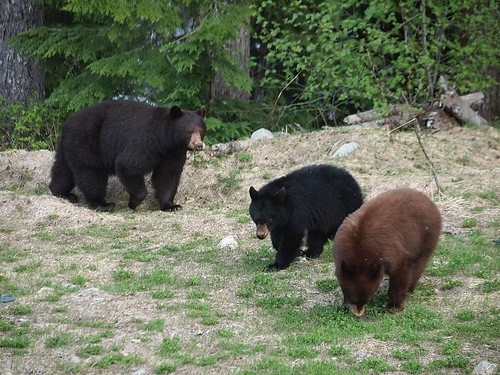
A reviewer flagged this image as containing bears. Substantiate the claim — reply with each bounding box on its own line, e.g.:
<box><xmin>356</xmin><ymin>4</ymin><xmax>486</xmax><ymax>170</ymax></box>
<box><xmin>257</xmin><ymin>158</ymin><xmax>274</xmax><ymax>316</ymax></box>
<box><xmin>49</xmin><ymin>100</ymin><xmax>207</xmax><ymax>213</ymax></box>
<box><xmin>333</xmin><ymin>188</ymin><xmax>442</xmax><ymax>319</ymax></box>
<box><xmin>248</xmin><ymin>164</ymin><xmax>364</xmax><ymax>272</ymax></box>
